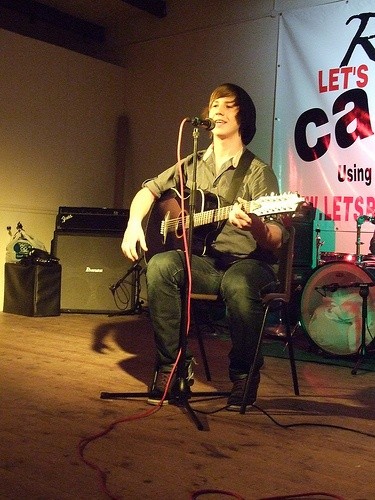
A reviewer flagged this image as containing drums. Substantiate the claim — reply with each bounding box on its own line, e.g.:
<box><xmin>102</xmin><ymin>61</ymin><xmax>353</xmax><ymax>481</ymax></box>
<box><xmin>299</xmin><ymin>260</ymin><xmax>375</xmax><ymax>355</ymax></box>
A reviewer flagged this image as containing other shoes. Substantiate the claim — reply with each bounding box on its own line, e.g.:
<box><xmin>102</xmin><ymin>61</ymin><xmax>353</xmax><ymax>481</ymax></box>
<box><xmin>147</xmin><ymin>358</ymin><xmax>193</xmax><ymax>405</ymax></box>
<box><xmin>226</xmin><ymin>370</ymin><xmax>261</xmax><ymax>409</ymax></box>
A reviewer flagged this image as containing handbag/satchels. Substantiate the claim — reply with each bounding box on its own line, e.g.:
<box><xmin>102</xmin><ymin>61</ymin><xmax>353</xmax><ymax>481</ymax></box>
<box><xmin>5</xmin><ymin>228</ymin><xmax>47</xmax><ymax>263</ymax></box>
<box><xmin>16</xmin><ymin>248</ymin><xmax>60</xmax><ymax>266</ymax></box>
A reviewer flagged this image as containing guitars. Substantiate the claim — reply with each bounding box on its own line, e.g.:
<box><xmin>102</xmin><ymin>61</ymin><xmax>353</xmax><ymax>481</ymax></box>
<box><xmin>144</xmin><ymin>187</ymin><xmax>306</xmax><ymax>263</ymax></box>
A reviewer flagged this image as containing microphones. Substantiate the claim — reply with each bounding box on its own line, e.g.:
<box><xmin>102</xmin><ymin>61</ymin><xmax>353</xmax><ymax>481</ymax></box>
<box><xmin>186</xmin><ymin>117</ymin><xmax>216</xmax><ymax>131</ymax></box>
<box><xmin>315</xmin><ymin>283</ymin><xmax>339</xmax><ymax>292</ymax></box>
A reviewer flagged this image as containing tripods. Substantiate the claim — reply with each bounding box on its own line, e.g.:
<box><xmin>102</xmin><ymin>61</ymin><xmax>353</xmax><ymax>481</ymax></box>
<box><xmin>109</xmin><ymin>256</ymin><xmax>149</xmax><ymax>317</ymax></box>
<box><xmin>325</xmin><ymin>282</ymin><xmax>375</xmax><ymax>375</ymax></box>
<box><xmin>101</xmin><ymin>125</ymin><xmax>230</xmax><ymax>430</ymax></box>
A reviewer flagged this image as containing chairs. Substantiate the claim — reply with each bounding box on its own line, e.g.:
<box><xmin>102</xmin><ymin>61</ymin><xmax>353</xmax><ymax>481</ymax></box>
<box><xmin>190</xmin><ymin>225</ymin><xmax>299</xmax><ymax>414</ymax></box>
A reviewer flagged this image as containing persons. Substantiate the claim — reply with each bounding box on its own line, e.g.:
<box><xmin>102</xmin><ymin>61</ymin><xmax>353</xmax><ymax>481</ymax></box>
<box><xmin>120</xmin><ymin>82</ymin><xmax>289</xmax><ymax>411</ymax></box>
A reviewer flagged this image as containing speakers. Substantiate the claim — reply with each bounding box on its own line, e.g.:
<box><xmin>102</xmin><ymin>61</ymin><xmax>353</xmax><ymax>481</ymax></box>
<box><xmin>52</xmin><ymin>230</ymin><xmax>149</xmax><ymax>314</ymax></box>
